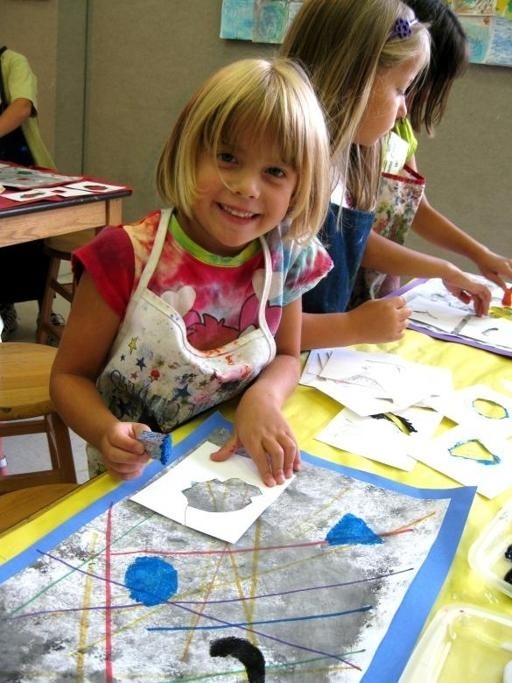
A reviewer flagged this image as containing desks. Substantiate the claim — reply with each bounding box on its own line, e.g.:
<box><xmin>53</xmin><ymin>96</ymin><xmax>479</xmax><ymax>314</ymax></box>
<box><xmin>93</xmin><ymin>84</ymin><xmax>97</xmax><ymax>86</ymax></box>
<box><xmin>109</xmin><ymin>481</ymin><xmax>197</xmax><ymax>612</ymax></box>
<box><xmin>1</xmin><ymin>278</ymin><xmax>512</xmax><ymax>682</ymax></box>
<box><xmin>0</xmin><ymin>157</ymin><xmax>133</xmax><ymax>249</ymax></box>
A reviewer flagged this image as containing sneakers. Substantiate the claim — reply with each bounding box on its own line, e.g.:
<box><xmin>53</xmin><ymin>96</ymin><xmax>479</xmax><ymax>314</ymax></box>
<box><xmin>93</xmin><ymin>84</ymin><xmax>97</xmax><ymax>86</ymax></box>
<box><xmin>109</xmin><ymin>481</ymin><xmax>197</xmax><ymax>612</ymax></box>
<box><xmin>1</xmin><ymin>305</ymin><xmax>20</xmax><ymax>341</ymax></box>
<box><xmin>37</xmin><ymin>313</ymin><xmax>65</xmax><ymax>347</ymax></box>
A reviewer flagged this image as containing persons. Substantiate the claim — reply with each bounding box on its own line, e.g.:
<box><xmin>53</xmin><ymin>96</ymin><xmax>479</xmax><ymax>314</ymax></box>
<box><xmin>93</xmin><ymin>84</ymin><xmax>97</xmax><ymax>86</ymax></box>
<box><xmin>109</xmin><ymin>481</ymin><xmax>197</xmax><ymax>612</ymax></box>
<box><xmin>48</xmin><ymin>54</ymin><xmax>335</xmax><ymax>486</ymax></box>
<box><xmin>0</xmin><ymin>43</ymin><xmax>66</xmax><ymax>343</ymax></box>
<box><xmin>275</xmin><ymin>0</ymin><xmax>494</xmax><ymax>351</ymax></box>
<box><xmin>347</xmin><ymin>0</ymin><xmax>512</xmax><ymax>317</ymax></box>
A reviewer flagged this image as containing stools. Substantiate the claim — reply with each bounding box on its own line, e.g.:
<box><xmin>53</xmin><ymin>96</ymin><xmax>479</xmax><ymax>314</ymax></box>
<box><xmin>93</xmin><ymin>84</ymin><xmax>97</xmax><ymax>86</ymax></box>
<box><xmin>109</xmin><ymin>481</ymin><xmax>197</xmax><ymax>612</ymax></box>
<box><xmin>1</xmin><ymin>340</ymin><xmax>77</xmax><ymax>493</ymax></box>
<box><xmin>1</xmin><ymin>484</ymin><xmax>78</xmax><ymax>535</ymax></box>
<box><xmin>35</xmin><ymin>210</ymin><xmax>137</xmax><ymax>344</ymax></box>
